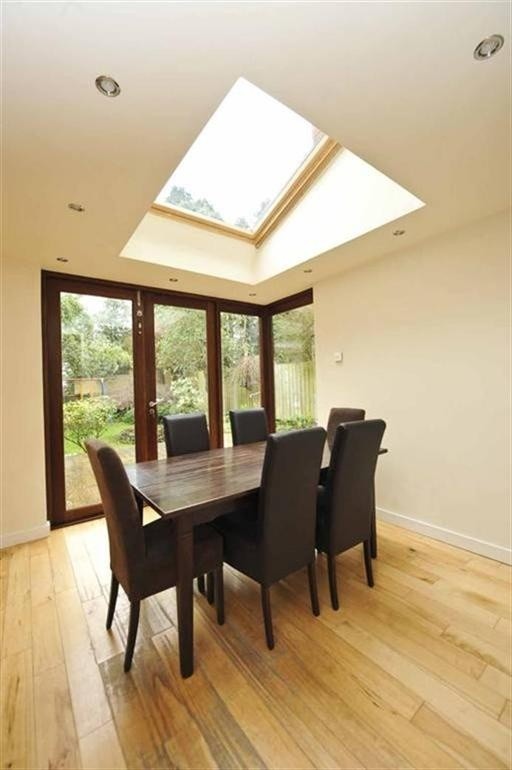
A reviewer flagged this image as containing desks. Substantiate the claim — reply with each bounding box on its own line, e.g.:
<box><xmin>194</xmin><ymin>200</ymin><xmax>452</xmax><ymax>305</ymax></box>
<box><xmin>124</xmin><ymin>439</ymin><xmax>388</xmax><ymax>679</ymax></box>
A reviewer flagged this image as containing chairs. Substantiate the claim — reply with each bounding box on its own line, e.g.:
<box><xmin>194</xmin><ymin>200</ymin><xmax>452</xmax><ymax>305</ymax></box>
<box><xmin>207</xmin><ymin>428</ymin><xmax>328</xmax><ymax>650</ymax></box>
<box><xmin>316</xmin><ymin>419</ymin><xmax>387</xmax><ymax>611</ymax></box>
<box><xmin>230</xmin><ymin>409</ymin><xmax>268</xmax><ymax>446</ymax></box>
<box><xmin>163</xmin><ymin>413</ymin><xmax>210</xmax><ymax>457</ymax></box>
<box><xmin>327</xmin><ymin>408</ymin><xmax>365</xmax><ymax>434</ymax></box>
<box><xmin>85</xmin><ymin>438</ymin><xmax>223</xmax><ymax>672</ymax></box>
